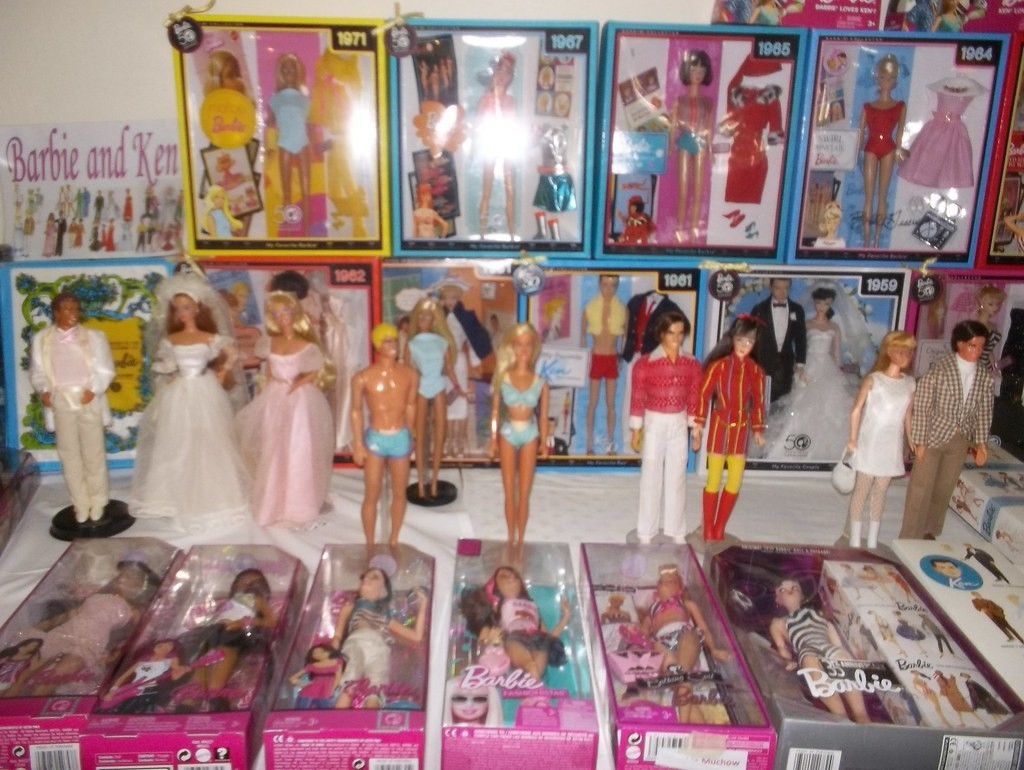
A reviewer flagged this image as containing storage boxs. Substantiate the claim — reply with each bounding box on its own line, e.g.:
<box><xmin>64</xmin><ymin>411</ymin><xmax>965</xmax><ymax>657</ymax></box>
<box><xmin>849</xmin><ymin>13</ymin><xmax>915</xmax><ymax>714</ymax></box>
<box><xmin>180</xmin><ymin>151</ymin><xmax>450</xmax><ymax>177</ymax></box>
<box><xmin>0</xmin><ymin>0</ymin><xmax>1024</xmax><ymax>769</ymax></box>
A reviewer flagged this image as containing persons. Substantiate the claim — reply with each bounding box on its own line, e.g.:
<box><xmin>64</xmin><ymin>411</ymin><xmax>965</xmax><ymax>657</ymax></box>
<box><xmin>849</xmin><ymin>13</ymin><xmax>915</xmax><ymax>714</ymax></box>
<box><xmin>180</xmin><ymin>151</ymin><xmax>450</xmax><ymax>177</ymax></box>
<box><xmin>0</xmin><ymin>50</ymin><xmax>1023</xmax><ymax>724</ymax></box>
